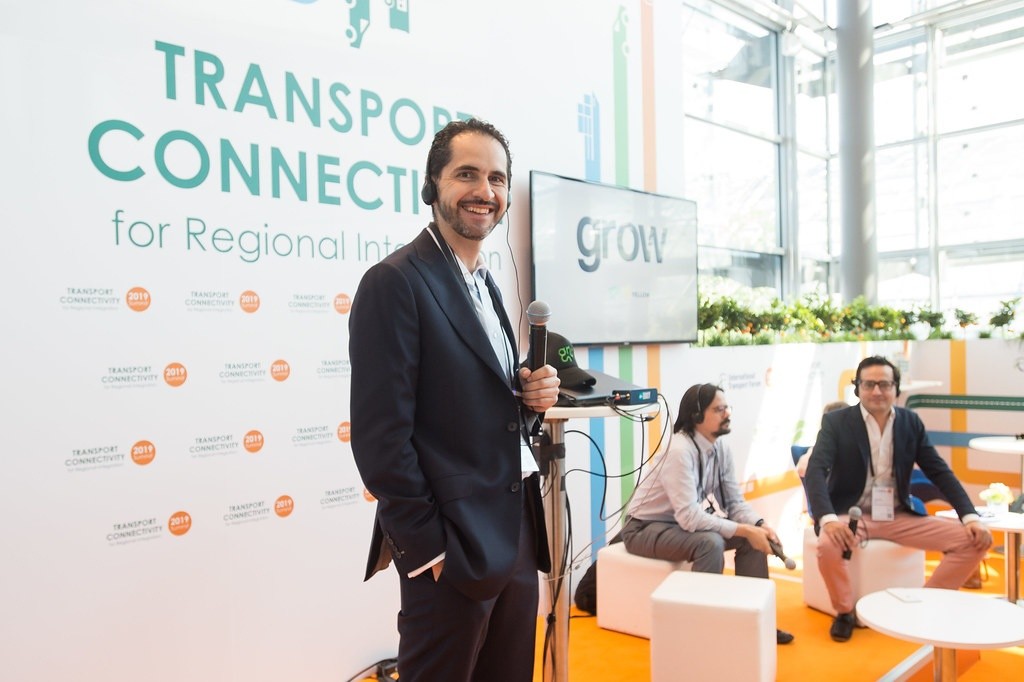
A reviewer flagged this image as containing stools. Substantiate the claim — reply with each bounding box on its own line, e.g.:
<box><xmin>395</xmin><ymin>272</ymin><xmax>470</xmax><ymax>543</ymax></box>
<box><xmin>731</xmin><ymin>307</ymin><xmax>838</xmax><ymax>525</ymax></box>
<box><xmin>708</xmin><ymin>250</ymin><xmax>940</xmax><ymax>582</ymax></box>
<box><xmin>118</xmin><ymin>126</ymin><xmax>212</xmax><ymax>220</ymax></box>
<box><xmin>649</xmin><ymin>571</ymin><xmax>776</xmax><ymax>682</ymax></box>
<box><xmin>803</xmin><ymin>525</ymin><xmax>926</xmax><ymax>628</ymax></box>
<box><xmin>596</xmin><ymin>541</ymin><xmax>694</xmax><ymax>638</ymax></box>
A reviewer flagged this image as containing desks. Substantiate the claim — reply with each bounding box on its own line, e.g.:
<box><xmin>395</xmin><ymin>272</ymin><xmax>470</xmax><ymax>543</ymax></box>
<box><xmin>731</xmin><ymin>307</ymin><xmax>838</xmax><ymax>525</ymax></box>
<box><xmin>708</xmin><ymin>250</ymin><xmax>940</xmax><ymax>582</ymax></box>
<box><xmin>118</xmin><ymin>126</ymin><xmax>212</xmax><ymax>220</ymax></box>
<box><xmin>856</xmin><ymin>588</ymin><xmax>1024</xmax><ymax>682</ymax></box>
<box><xmin>968</xmin><ymin>436</ymin><xmax>1024</xmax><ymax>496</ymax></box>
<box><xmin>529</xmin><ymin>401</ymin><xmax>656</xmax><ymax>682</ymax></box>
<box><xmin>935</xmin><ymin>505</ymin><xmax>1024</xmax><ymax>605</ymax></box>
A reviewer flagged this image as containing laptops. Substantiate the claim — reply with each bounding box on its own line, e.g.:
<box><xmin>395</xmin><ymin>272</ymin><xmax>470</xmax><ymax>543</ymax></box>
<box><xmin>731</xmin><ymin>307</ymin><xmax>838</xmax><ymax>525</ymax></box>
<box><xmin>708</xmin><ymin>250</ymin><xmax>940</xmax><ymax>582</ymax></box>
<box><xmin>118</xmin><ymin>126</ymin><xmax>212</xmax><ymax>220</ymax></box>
<box><xmin>553</xmin><ymin>368</ymin><xmax>645</xmax><ymax>407</ymax></box>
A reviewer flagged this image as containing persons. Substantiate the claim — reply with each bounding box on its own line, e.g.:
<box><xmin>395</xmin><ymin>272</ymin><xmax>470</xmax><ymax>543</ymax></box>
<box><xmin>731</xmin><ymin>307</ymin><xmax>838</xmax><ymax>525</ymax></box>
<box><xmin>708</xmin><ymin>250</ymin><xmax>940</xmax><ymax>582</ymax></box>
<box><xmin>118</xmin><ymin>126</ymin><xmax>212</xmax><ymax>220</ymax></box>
<box><xmin>622</xmin><ymin>383</ymin><xmax>794</xmax><ymax>645</ymax></box>
<box><xmin>347</xmin><ymin>118</ymin><xmax>561</xmax><ymax>682</ymax></box>
<box><xmin>804</xmin><ymin>356</ymin><xmax>993</xmax><ymax>641</ymax></box>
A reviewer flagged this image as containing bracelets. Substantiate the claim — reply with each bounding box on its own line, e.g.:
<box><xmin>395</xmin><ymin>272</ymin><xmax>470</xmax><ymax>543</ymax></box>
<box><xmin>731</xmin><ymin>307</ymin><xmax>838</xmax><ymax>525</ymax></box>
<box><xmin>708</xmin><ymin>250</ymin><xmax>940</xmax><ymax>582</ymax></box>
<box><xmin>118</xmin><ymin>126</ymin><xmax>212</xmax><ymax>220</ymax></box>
<box><xmin>755</xmin><ymin>518</ymin><xmax>764</xmax><ymax>526</ymax></box>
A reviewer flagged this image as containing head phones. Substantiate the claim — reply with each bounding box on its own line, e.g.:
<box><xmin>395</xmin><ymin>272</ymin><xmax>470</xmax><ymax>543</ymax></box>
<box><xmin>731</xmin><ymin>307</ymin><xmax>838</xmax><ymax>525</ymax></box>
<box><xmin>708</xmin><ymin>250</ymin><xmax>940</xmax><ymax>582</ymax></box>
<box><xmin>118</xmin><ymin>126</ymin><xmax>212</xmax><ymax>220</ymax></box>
<box><xmin>853</xmin><ymin>360</ymin><xmax>902</xmax><ymax>398</ymax></box>
<box><xmin>420</xmin><ymin>148</ymin><xmax>512</xmax><ymax>213</ymax></box>
<box><xmin>692</xmin><ymin>384</ymin><xmax>705</xmax><ymax>424</ymax></box>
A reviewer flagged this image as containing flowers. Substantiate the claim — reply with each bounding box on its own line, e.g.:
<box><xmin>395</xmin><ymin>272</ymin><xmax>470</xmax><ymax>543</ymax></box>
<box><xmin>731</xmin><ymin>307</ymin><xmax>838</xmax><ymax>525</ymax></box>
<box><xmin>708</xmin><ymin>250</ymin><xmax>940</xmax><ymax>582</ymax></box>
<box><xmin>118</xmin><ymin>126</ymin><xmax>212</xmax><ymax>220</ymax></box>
<box><xmin>697</xmin><ymin>292</ymin><xmax>1022</xmax><ymax>348</ymax></box>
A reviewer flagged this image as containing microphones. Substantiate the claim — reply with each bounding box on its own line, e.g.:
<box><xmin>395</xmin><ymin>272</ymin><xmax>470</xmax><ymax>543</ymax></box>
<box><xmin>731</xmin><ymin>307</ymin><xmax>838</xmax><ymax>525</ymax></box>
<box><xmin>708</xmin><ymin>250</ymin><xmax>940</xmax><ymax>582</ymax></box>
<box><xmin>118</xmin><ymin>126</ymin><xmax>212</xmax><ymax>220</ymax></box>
<box><xmin>526</xmin><ymin>300</ymin><xmax>552</xmax><ymax>383</ymax></box>
<box><xmin>841</xmin><ymin>506</ymin><xmax>862</xmax><ymax>561</ymax></box>
<box><xmin>766</xmin><ymin>537</ymin><xmax>796</xmax><ymax>570</ymax></box>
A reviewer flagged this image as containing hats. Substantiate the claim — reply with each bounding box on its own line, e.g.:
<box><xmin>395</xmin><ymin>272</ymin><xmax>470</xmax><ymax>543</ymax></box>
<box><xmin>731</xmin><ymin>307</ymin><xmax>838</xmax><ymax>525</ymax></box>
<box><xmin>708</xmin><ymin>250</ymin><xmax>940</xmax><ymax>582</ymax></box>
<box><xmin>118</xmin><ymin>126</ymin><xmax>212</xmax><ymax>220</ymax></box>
<box><xmin>526</xmin><ymin>329</ymin><xmax>596</xmax><ymax>388</ymax></box>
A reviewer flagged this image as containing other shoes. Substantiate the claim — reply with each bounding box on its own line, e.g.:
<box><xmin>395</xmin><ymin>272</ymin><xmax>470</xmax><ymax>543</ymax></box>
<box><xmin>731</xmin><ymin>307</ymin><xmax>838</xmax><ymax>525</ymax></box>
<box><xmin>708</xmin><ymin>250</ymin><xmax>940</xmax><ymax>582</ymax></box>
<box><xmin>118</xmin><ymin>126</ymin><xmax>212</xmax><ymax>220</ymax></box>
<box><xmin>776</xmin><ymin>629</ymin><xmax>794</xmax><ymax>645</ymax></box>
<box><xmin>830</xmin><ymin>611</ymin><xmax>856</xmax><ymax>642</ymax></box>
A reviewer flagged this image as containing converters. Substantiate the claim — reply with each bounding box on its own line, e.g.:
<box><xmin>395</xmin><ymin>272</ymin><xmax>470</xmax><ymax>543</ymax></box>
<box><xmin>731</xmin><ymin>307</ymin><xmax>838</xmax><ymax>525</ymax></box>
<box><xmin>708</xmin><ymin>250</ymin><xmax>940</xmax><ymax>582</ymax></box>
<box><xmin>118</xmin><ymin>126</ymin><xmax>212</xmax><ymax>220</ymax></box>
<box><xmin>612</xmin><ymin>388</ymin><xmax>658</xmax><ymax>405</ymax></box>
<box><xmin>531</xmin><ymin>430</ymin><xmax>549</xmax><ymax>474</ymax></box>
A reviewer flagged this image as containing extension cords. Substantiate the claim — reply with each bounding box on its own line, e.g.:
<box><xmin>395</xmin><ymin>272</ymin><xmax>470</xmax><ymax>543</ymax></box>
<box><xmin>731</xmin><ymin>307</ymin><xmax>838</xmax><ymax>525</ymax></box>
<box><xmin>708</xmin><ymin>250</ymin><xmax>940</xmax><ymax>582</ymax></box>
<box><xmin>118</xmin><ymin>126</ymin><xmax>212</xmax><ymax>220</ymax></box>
<box><xmin>376</xmin><ymin>658</ymin><xmax>400</xmax><ymax>677</ymax></box>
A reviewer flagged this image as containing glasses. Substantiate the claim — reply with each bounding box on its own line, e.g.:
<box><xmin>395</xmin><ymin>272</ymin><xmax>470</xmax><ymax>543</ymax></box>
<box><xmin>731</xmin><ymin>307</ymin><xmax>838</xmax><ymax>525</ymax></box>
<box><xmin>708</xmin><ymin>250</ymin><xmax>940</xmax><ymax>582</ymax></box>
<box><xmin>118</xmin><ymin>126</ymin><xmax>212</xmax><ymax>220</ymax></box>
<box><xmin>704</xmin><ymin>406</ymin><xmax>732</xmax><ymax>417</ymax></box>
<box><xmin>856</xmin><ymin>379</ymin><xmax>897</xmax><ymax>391</ymax></box>
